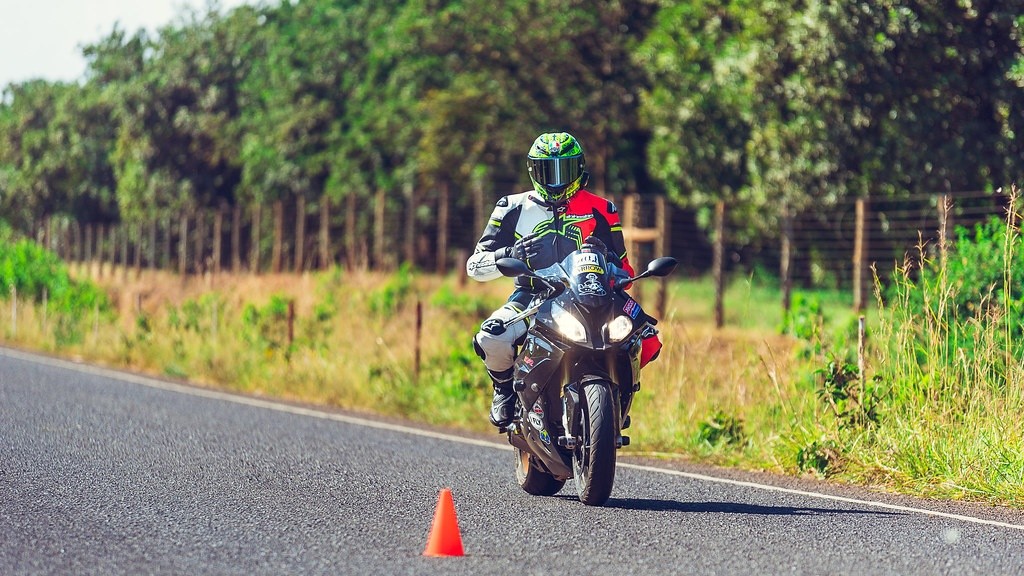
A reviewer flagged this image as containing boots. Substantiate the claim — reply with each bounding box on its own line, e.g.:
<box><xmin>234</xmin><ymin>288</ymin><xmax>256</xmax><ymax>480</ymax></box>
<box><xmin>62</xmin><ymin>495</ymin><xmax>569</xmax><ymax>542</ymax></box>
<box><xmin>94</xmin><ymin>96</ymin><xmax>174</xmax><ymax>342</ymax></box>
<box><xmin>486</xmin><ymin>365</ymin><xmax>514</xmax><ymax>427</ymax></box>
<box><xmin>621</xmin><ymin>415</ymin><xmax>630</xmax><ymax>429</ymax></box>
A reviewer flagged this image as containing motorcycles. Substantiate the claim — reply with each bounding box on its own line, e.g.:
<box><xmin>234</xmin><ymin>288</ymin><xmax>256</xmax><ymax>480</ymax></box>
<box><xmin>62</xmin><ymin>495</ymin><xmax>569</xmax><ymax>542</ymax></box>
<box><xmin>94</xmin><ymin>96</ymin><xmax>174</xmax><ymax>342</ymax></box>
<box><xmin>498</xmin><ymin>244</ymin><xmax>677</xmax><ymax>506</ymax></box>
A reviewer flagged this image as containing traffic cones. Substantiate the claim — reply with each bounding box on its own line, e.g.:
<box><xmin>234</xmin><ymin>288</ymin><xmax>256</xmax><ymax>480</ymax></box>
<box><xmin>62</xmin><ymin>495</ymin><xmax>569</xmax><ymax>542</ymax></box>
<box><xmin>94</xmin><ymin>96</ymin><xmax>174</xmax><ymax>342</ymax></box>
<box><xmin>423</xmin><ymin>488</ymin><xmax>468</xmax><ymax>560</ymax></box>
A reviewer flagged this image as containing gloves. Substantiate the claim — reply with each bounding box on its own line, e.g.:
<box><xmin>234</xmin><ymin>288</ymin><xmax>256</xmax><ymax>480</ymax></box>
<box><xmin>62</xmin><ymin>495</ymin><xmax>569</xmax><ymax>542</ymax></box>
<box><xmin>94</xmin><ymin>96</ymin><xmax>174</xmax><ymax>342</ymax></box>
<box><xmin>582</xmin><ymin>236</ymin><xmax>622</xmax><ymax>268</ymax></box>
<box><xmin>494</xmin><ymin>233</ymin><xmax>544</xmax><ymax>262</ymax></box>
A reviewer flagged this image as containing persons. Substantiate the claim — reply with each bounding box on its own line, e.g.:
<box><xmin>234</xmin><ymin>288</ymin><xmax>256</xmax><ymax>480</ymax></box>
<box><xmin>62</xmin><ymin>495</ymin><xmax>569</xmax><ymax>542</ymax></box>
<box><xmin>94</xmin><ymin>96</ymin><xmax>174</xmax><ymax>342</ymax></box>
<box><xmin>466</xmin><ymin>132</ymin><xmax>663</xmax><ymax>427</ymax></box>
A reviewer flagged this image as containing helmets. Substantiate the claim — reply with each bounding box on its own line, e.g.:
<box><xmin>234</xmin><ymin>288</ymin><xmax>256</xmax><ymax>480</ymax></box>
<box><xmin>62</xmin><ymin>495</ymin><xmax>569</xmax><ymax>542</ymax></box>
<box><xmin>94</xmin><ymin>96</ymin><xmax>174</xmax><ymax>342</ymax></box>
<box><xmin>526</xmin><ymin>132</ymin><xmax>590</xmax><ymax>203</ymax></box>
